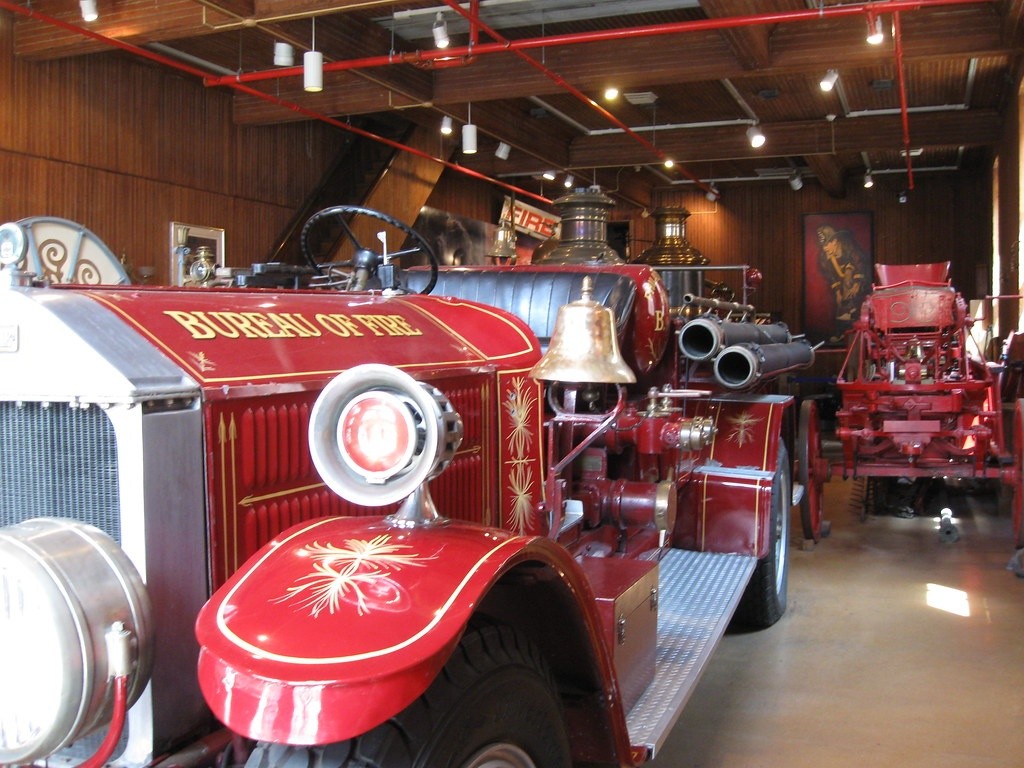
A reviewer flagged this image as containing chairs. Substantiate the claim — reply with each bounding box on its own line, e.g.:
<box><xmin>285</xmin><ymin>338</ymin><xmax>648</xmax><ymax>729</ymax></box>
<box><xmin>873</xmin><ymin>259</ymin><xmax>951</xmax><ymax>381</ymax></box>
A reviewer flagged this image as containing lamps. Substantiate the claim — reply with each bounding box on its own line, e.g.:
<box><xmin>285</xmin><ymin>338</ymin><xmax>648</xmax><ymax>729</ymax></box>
<box><xmin>440</xmin><ymin>117</ymin><xmax>453</xmax><ymax>134</ymax></box>
<box><xmin>564</xmin><ymin>175</ymin><xmax>574</xmax><ymax>188</ymax></box>
<box><xmin>745</xmin><ymin>126</ymin><xmax>767</xmax><ymax>149</ymax></box>
<box><xmin>461</xmin><ymin>102</ymin><xmax>477</xmax><ymax>154</ymax></box>
<box><xmin>494</xmin><ymin>143</ymin><xmax>511</xmax><ymax>160</ymax></box>
<box><xmin>303</xmin><ymin>17</ymin><xmax>323</xmax><ymax>93</ymax></box>
<box><xmin>273</xmin><ymin>41</ymin><xmax>294</xmax><ymax>67</ymax></box>
<box><xmin>544</xmin><ymin>171</ymin><xmax>556</xmax><ymax>181</ymax></box>
<box><xmin>819</xmin><ymin>67</ymin><xmax>839</xmax><ymax>91</ymax></box>
<box><xmin>864</xmin><ymin>176</ymin><xmax>874</xmax><ymax>188</ymax></box>
<box><xmin>788</xmin><ymin>175</ymin><xmax>803</xmax><ymax>190</ymax></box>
<box><xmin>79</xmin><ymin>0</ymin><xmax>99</xmax><ymax>22</ymax></box>
<box><xmin>431</xmin><ymin>12</ymin><xmax>450</xmax><ymax>49</ymax></box>
<box><xmin>706</xmin><ymin>183</ymin><xmax>719</xmax><ymax>203</ymax></box>
<box><xmin>865</xmin><ymin>15</ymin><xmax>883</xmax><ymax>44</ymax></box>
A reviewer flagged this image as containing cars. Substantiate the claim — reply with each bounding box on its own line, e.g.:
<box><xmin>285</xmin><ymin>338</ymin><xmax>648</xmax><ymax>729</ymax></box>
<box><xmin>796</xmin><ymin>260</ymin><xmax>1023</xmax><ymax>540</ymax></box>
<box><xmin>0</xmin><ymin>184</ymin><xmax>828</xmax><ymax>768</ymax></box>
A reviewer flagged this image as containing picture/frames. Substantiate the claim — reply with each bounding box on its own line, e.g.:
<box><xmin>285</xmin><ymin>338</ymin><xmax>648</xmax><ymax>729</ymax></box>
<box><xmin>800</xmin><ymin>210</ymin><xmax>875</xmax><ymax>349</ymax></box>
<box><xmin>169</xmin><ymin>221</ymin><xmax>226</xmax><ymax>287</ymax></box>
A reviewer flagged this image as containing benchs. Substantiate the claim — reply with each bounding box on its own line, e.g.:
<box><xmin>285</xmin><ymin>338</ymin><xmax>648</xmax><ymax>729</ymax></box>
<box><xmin>405</xmin><ymin>265</ymin><xmax>638</xmax><ymax>406</ymax></box>
